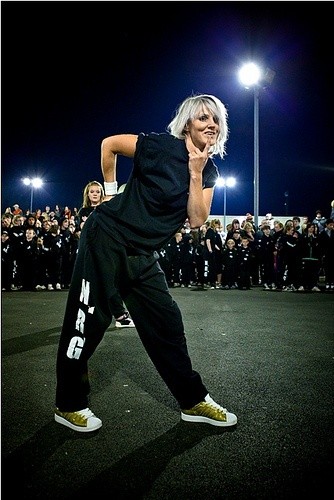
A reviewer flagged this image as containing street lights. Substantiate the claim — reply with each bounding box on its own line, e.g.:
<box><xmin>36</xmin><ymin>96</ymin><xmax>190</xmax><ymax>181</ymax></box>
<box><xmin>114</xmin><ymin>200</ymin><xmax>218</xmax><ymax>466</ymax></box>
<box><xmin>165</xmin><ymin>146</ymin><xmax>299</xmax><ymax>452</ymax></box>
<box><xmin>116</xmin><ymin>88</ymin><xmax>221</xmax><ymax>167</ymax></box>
<box><xmin>22</xmin><ymin>178</ymin><xmax>44</xmax><ymax>214</ymax></box>
<box><xmin>215</xmin><ymin>177</ymin><xmax>235</xmax><ymax>232</ymax></box>
<box><xmin>238</xmin><ymin>63</ymin><xmax>276</xmax><ymax>226</ymax></box>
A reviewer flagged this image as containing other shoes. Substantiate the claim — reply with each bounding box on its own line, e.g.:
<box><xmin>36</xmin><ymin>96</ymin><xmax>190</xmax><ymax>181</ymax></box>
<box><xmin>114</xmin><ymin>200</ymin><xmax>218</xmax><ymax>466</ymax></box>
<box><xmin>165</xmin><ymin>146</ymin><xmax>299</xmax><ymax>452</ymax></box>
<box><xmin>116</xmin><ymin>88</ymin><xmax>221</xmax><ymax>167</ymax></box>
<box><xmin>312</xmin><ymin>286</ymin><xmax>320</xmax><ymax>291</ymax></box>
<box><xmin>297</xmin><ymin>286</ymin><xmax>305</xmax><ymax>291</ymax></box>
<box><xmin>48</xmin><ymin>284</ymin><xmax>54</xmax><ymax>291</ymax></box>
<box><xmin>56</xmin><ymin>282</ymin><xmax>62</xmax><ymax>289</ymax></box>
<box><xmin>115</xmin><ymin>311</ymin><xmax>135</xmax><ymax>328</ymax></box>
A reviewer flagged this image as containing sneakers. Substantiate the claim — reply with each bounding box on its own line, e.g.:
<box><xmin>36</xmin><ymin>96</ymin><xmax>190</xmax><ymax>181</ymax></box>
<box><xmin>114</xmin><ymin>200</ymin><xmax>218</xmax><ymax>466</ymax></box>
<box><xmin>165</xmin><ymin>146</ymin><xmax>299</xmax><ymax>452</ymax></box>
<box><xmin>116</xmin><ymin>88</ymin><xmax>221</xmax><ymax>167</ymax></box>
<box><xmin>180</xmin><ymin>392</ymin><xmax>238</xmax><ymax>427</ymax></box>
<box><xmin>54</xmin><ymin>407</ymin><xmax>102</xmax><ymax>432</ymax></box>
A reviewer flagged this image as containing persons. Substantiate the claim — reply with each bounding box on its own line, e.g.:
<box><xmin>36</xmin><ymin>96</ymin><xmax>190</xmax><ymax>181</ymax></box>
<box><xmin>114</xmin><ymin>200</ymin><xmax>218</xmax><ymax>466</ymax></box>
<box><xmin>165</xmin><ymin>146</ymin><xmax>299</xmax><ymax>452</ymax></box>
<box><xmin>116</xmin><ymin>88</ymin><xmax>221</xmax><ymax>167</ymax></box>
<box><xmin>1</xmin><ymin>202</ymin><xmax>78</xmax><ymax>291</ymax></box>
<box><xmin>77</xmin><ymin>181</ymin><xmax>135</xmax><ymax>328</ymax></box>
<box><xmin>157</xmin><ymin>199</ymin><xmax>334</xmax><ymax>294</ymax></box>
<box><xmin>55</xmin><ymin>95</ymin><xmax>238</xmax><ymax>432</ymax></box>
